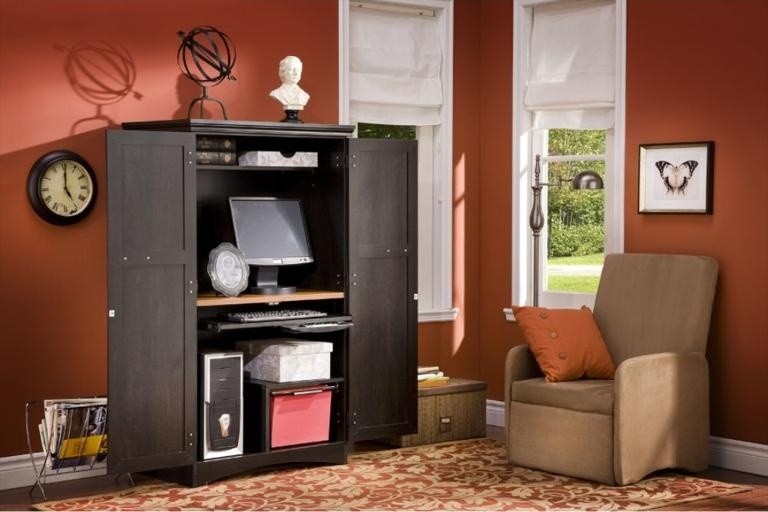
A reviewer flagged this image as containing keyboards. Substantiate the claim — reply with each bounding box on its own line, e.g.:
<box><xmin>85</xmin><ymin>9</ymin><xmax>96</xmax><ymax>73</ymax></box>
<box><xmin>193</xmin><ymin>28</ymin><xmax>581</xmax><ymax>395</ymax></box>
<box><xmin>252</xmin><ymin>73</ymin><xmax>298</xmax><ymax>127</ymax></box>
<box><xmin>216</xmin><ymin>310</ymin><xmax>328</xmax><ymax>322</ymax></box>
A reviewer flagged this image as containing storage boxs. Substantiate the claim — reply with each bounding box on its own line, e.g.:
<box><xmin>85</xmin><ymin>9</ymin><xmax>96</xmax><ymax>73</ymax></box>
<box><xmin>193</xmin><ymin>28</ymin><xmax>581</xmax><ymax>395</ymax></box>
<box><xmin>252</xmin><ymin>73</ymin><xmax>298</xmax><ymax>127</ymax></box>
<box><xmin>390</xmin><ymin>379</ymin><xmax>488</xmax><ymax>449</ymax></box>
<box><xmin>236</xmin><ymin>337</ymin><xmax>333</xmax><ymax>384</ymax></box>
<box><xmin>239</xmin><ymin>151</ymin><xmax>318</xmax><ymax>167</ymax></box>
<box><xmin>270</xmin><ymin>386</ymin><xmax>335</xmax><ymax>448</ymax></box>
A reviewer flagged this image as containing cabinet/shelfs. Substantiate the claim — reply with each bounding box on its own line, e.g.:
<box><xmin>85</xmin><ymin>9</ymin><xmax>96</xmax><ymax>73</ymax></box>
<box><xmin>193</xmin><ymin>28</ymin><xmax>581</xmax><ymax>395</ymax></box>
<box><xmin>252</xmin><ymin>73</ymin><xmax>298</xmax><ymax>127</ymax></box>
<box><xmin>105</xmin><ymin>118</ymin><xmax>420</xmax><ymax>488</ymax></box>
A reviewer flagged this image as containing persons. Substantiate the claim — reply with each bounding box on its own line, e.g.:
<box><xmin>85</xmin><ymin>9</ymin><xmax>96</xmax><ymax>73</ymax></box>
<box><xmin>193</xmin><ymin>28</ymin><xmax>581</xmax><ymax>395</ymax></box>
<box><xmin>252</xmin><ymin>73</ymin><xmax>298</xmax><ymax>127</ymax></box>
<box><xmin>269</xmin><ymin>56</ymin><xmax>310</xmax><ymax>110</ymax></box>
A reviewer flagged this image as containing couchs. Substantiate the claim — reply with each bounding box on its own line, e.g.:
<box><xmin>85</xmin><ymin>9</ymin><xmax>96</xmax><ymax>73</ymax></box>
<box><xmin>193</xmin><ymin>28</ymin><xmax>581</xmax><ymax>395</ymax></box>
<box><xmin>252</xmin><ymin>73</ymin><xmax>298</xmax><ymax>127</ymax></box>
<box><xmin>504</xmin><ymin>254</ymin><xmax>719</xmax><ymax>487</ymax></box>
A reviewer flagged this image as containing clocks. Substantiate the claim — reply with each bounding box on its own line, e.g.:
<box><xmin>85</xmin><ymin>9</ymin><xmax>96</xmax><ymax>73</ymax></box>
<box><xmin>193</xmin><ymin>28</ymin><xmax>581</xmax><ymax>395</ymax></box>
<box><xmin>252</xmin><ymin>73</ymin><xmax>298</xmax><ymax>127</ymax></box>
<box><xmin>26</xmin><ymin>149</ymin><xmax>98</xmax><ymax>226</ymax></box>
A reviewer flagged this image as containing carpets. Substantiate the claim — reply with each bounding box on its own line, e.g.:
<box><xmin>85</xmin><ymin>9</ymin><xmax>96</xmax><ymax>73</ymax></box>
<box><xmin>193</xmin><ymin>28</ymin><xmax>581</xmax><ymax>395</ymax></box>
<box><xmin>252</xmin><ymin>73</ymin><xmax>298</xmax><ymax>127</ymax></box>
<box><xmin>29</xmin><ymin>437</ymin><xmax>753</xmax><ymax>511</ymax></box>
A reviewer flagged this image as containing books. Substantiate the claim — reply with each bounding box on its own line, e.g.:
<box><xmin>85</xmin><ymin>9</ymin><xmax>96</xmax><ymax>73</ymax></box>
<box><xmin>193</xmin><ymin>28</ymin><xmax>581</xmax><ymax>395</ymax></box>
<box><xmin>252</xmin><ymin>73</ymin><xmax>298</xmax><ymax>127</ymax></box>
<box><xmin>39</xmin><ymin>397</ymin><xmax>108</xmax><ymax>469</ymax></box>
<box><xmin>417</xmin><ymin>366</ymin><xmax>442</xmax><ymax>381</ymax></box>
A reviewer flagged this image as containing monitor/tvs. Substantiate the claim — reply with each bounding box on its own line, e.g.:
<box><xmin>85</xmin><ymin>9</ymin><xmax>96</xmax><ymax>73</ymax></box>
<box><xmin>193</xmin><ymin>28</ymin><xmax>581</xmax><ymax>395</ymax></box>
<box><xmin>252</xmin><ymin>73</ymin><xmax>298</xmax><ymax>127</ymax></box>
<box><xmin>228</xmin><ymin>196</ymin><xmax>316</xmax><ymax>295</ymax></box>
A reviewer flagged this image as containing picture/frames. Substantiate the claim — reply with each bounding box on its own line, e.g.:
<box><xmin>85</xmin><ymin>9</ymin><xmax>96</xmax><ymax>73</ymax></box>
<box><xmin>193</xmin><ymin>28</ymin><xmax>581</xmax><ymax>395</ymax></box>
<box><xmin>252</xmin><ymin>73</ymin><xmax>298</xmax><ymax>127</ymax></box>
<box><xmin>208</xmin><ymin>242</ymin><xmax>250</xmax><ymax>297</ymax></box>
<box><xmin>638</xmin><ymin>141</ymin><xmax>715</xmax><ymax>214</ymax></box>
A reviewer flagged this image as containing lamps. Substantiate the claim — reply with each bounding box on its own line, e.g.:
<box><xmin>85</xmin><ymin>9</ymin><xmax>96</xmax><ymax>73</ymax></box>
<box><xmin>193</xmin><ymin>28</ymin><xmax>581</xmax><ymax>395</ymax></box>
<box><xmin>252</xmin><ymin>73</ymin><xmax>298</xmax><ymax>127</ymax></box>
<box><xmin>529</xmin><ymin>154</ymin><xmax>604</xmax><ymax>307</ymax></box>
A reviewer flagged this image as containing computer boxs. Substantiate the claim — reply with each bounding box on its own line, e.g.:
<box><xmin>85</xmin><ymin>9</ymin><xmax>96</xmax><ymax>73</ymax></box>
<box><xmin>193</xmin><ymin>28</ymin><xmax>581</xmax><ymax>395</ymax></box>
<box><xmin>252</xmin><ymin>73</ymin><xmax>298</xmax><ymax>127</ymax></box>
<box><xmin>197</xmin><ymin>350</ymin><xmax>244</xmax><ymax>461</ymax></box>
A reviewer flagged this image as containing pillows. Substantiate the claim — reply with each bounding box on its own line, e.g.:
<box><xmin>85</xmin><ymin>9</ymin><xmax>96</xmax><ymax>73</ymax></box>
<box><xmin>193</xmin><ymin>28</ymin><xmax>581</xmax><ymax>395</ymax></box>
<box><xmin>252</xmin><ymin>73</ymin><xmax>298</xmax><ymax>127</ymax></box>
<box><xmin>512</xmin><ymin>305</ymin><xmax>616</xmax><ymax>384</ymax></box>
